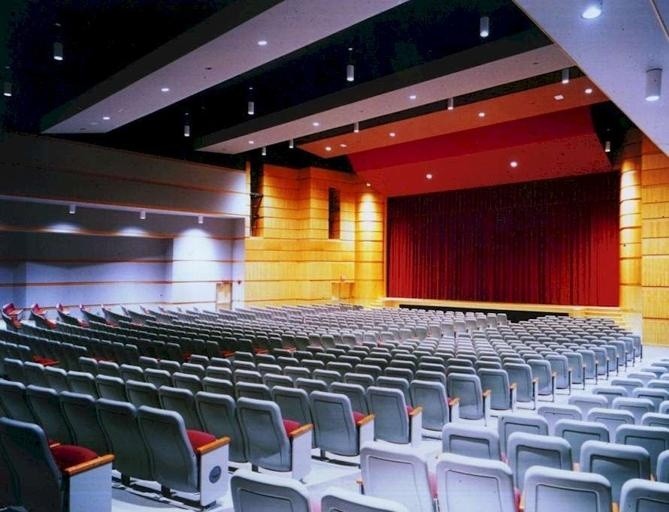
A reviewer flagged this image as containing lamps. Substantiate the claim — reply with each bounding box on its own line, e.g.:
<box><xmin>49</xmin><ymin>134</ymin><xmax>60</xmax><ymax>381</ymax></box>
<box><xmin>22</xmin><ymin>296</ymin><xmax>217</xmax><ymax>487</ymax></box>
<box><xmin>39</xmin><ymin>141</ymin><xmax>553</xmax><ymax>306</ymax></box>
<box><xmin>446</xmin><ymin>94</ymin><xmax>455</xmax><ymax>111</ymax></box>
<box><xmin>183</xmin><ymin>124</ymin><xmax>190</xmax><ymax>138</ymax></box>
<box><xmin>353</xmin><ymin>119</ymin><xmax>360</xmax><ymax>134</ymax></box>
<box><xmin>246</xmin><ymin>99</ymin><xmax>256</xmax><ymax>116</ymax></box>
<box><xmin>287</xmin><ymin>138</ymin><xmax>295</xmax><ymax>150</ymax></box>
<box><xmin>561</xmin><ymin>65</ymin><xmax>571</xmax><ymax>85</ymax></box>
<box><xmin>260</xmin><ymin>145</ymin><xmax>268</xmax><ymax>157</ymax></box>
<box><xmin>644</xmin><ymin>66</ymin><xmax>662</xmax><ymax>104</ymax></box>
<box><xmin>346</xmin><ymin>61</ymin><xmax>355</xmax><ymax>82</ymax></box>
<box><xmin>52</xmin><ymin>37</ymin><xmax>65</xmax><ymax>63</ymax></box>
<box><xmin>478</xmin><ymin>12</ymin><xmax>489</xmax><ymax>39</ymax></box>
<box><xmin>197</xmin><ymin>213</ymin><xmax>205</xmax><ymax>226</ymax></box>
<box><xmin>67</xmin><ymin>201</ymin><xmax>77</xmax><ymax>215</ymax></box>
<box><xmin>603</xmin><ymin>140</ymin><xmax>612</xmax><ymax>153</ymax></box>
<box><xmin>139</xmin><ymin>208</ymin><xmax>147</xmax><ymax>222</ymax></box>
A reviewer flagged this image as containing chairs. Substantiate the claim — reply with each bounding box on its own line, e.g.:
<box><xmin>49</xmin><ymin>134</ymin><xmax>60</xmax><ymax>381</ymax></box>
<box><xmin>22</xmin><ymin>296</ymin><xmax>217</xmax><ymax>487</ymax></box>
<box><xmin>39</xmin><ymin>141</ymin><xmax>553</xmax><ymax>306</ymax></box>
<box><xmin>0</xmin><ymin>293</ymin><xmax>668</xmax><ymax>512</ymax></box>
